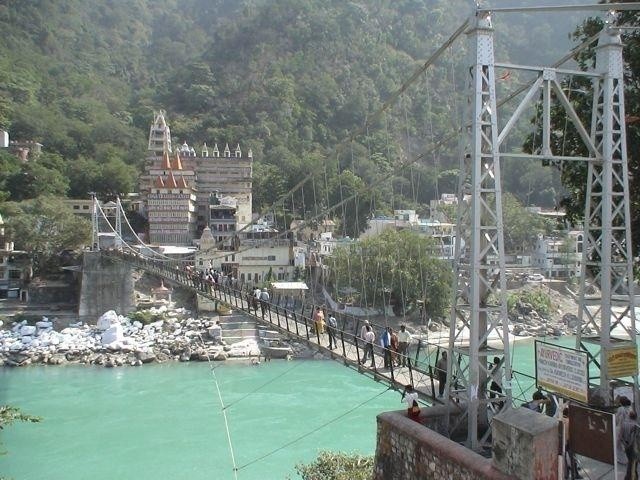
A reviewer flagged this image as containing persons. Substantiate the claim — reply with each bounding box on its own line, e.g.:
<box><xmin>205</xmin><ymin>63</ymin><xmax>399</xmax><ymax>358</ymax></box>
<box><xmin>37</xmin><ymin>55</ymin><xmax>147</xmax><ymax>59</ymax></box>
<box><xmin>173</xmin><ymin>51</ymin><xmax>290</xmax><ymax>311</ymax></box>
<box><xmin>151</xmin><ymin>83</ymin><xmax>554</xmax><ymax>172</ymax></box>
<box><xmin>247</xmin><ymin>287</ymin><xmax>262</xmax><ymax>300</ymax></box>
<box><xmin>181</xmin><ymin>262</ymin><xmax>234</xmax><ymax>291</ymax></box>
<box><xmin>326</xmin><ymin>313</ymin><xmax>338</xmax><ymax>350</ymax></box>
<box><xmin>615</xmin><ymin>395</ymin><xmax>634</xmax><ymax>426</ymax></box>
<box><xmin>436</xmin><ymin>351</ymin><xmax>447</xmax><ymax>396</ymax></box>
<box><xmin>361</xmin><ymin>320</ymin><xmax>372</xmax><ymax>360</ymax></box>
<box><xmin>400</xmin><ymin>385</ymin><xmax>419</xmax><ymax>422</ymax></box>
<box><xmin>313</xmin><ymin>307</ymin><xmax>326</xmax><ymax>335</ymax></box>
<box><xmin>260</xmin><ymin>288</ymin><xmax>270</xmax><ymax>317</ymax></box>
<box><xmin>397</xmin><ymin>325</ymin><xmax>412</xmax><ymax>367</ymax></box>
<box><xmin>619</xmin><ymin>410</ymin><xmax>640</xmax><ymax>480</ymax></box>
<box><xmin>527</xmin><ymin>391</ymin><xmax>552</xmax><ymax>412</ymax></box>
<box><xmin>359</xmin><ymin>325</ymin><xmax>376</xmax><ymax>367</ymax></box>
<box><xmin>488</xmin><ymin>357</ymin><xmax>504</xmax><ymax>409</ymax></box>
<box><xmin>387</xmin><ymin>328</ymin><xmax>399</xmax><ymax>367</ymax></box>
<box><xmin>380</xmin><ymin>326</ymin><xmax>393</xmax><ymax>368</ymax></box>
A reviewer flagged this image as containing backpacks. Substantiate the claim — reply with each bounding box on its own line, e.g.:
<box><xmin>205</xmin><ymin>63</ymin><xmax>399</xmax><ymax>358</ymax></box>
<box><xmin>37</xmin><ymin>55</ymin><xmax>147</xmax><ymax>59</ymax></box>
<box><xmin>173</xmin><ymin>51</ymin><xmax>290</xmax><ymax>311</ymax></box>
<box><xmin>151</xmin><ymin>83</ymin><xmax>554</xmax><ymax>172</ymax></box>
<box><xmin>411</xmin><ymin>399</ymin><xmax>420</xmax><ymax>417</ymax></box>
